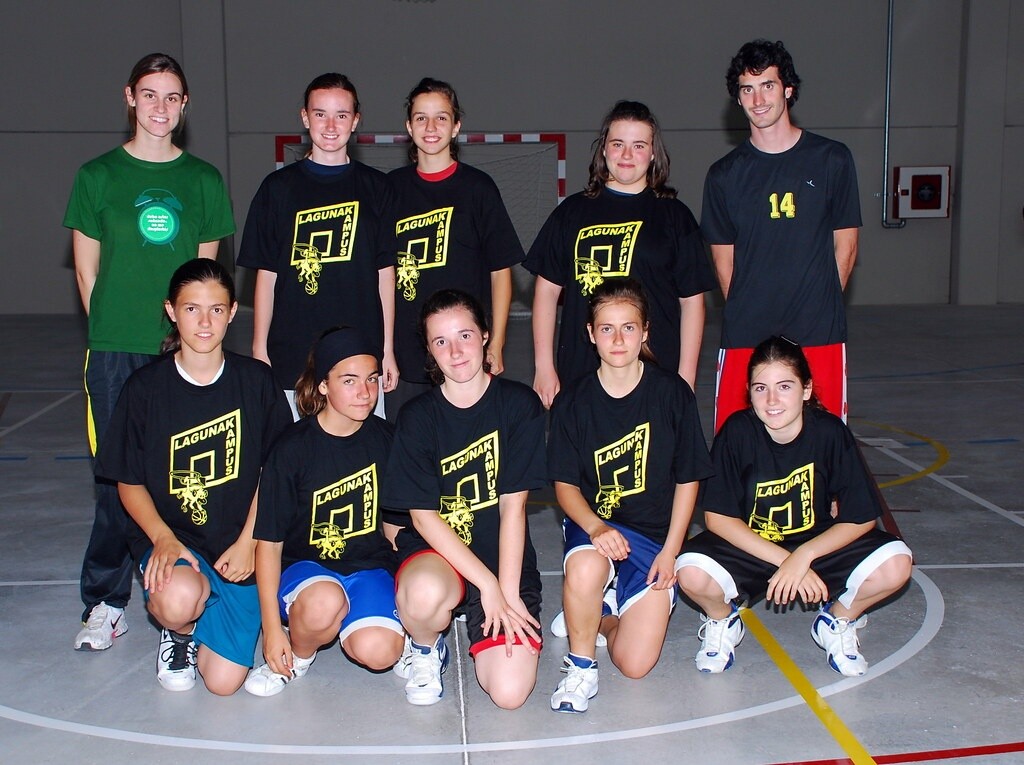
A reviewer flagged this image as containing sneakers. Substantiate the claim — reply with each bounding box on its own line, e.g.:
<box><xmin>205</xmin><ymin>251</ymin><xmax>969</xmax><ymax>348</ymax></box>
<box><xmin>810</xmin><ymin>603</ymin><xmax>868</xmax><ymax>678</ymax></box>
<box><xmin>74</xmin><ymin>601</ymin><xmax>128</xmax><ymax>652</ymax></box>
<box><xmin>404</xmin><ymin>635</ymin><xmax>449</xmax><ymax>705</ymax></box>
<box><xmin>594</xmin><ymin>633</ymin><xmax>608</xmax><ymax>647</ymax></box>
<box><xmin>395</xmin><ymin>633</ymin><xmax>413</xmax><ymax>679</ymax></box>
<box><xmin>695</xmin><ymin>604</ymin><xmax>745</xmax><ymax>672</ymax></box>
<box><xmin>157</xmin><ymin>621</ymin><xmax>197</xmax><ymax>692</ymax></box>
<box><xmin>550</xmin><ymin>589</ymin><xmax>617</xmax><ymax>638</ymax></box>
<box><xmin>551</xmin><ymin>657</ymin><xmax>600</xmax><ymax>712</ymax></box>
<box><xmin>244</xmin><ymin>649</ymin><xmax>319</xmax><ymax>698</ymax></box>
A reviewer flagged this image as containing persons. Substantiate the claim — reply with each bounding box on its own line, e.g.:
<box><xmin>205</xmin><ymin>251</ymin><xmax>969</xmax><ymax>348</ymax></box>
<box><xmin>670</xmin><ymin>334</ymin><xmax>912</xmax><ymax>680</ymax></box>
<box><xmin>81</xmin><ymin>256</ymin><xmax>294</xmax><ymax>703</ymax></box>
<box><xmin>690</xmin><ymin>36</ymin><xmax>865</xmax><ymax>488</ymax></box>
<box><xmin>58</xmin><ymin>49</ymin><xmax>237</xmax><ymax>653</ymax></box>
<box><xmin>520</xmin><ymin>98</ymin><xmax>717</xmax><ymax>650</ymax></box>
<box><xmin>380</xmin><ymin>288</ymin><xmax>551</xmax><ymax>712</ymax></box>
<box><xmin>373</xmin><ymin>72</ymin><xmax>524</xmax><ymax>432</ymax></box>
<box><xmin>547</xmin><ymin>276</ymin><xmax>715</xmax><ymax>719</ymax></box>
<box><xmin>241</xmin><ymin>325</ymin><xmax>408</xmax><ymax>700</ymax></box>
<box><xmin>228</xmin><ymin>68</ymin><xmax>402</xmax><ymax>454</ymax></box>
